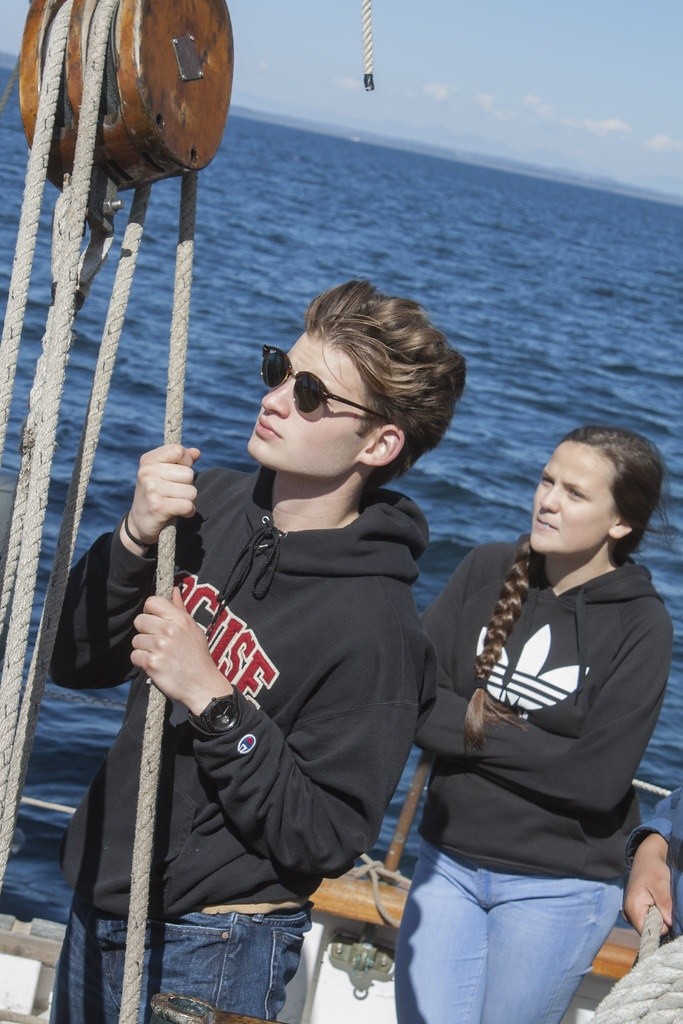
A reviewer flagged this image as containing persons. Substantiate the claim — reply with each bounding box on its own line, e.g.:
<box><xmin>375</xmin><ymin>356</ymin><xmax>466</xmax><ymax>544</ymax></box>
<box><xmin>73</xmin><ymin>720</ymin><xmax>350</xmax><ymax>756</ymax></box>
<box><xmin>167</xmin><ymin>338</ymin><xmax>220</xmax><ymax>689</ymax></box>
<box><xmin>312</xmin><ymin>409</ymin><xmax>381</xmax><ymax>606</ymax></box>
<box><xmin>622</xmin><ymin>783</ymin><xmax>683</xmax><ymax>965</ymax></box>
<box><xmin>393</xmin><ymin>425</ymin><xmax>675</xmax><ymax>1023</ymax></box>
<box><xmin>47</xmin><ymin>276</ymin><xmax>467</xmax><ymax>1023</ymax></box>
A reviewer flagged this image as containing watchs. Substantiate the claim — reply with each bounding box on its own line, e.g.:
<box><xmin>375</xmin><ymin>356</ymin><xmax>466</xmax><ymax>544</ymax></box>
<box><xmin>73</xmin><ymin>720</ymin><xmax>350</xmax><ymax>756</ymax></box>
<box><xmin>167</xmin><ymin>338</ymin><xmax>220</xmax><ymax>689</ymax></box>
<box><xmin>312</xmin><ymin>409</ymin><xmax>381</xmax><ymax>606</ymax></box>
<box><xmin>190</xmin><ymin>686</ymin><xmax>241</xmax><ymax>733</ymax></box>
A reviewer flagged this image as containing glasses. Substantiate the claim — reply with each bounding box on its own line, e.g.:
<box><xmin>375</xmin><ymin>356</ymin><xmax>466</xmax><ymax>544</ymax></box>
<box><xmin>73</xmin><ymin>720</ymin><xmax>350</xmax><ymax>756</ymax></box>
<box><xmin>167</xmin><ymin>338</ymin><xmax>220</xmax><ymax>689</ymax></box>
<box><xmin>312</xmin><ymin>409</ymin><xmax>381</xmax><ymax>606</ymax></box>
<box><xmin>259</xmin><ymin>344</ymin><xmax>390</xmax><ymax>419</ymax></box>
